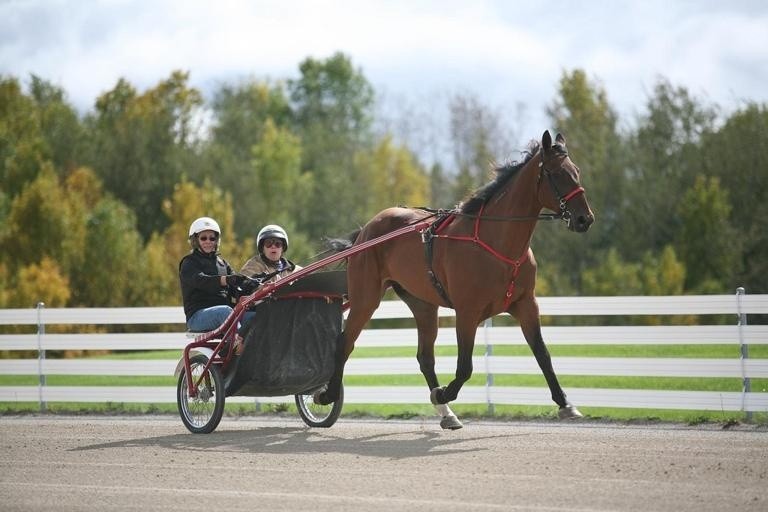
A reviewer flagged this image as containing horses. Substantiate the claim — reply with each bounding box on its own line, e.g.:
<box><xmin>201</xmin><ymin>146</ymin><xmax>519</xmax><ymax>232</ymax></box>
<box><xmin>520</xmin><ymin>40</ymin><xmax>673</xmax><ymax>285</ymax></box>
<box><xmin>311</xmin><ymin>128</ymin><xmax>597</xmax><ymax>431</ymax></box>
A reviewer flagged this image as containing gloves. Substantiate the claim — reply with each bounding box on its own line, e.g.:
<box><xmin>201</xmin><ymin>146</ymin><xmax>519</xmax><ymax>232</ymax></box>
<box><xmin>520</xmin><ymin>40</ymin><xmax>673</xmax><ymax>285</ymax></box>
<box><xmin>242</xmin><ymin>276</ymin><xmax>260</xmax><ymax>290</ymax></box>
<box><xmin>226</xmin><ymin>274</ymin><xmax>244</xmax><ymax>289</ymax></box>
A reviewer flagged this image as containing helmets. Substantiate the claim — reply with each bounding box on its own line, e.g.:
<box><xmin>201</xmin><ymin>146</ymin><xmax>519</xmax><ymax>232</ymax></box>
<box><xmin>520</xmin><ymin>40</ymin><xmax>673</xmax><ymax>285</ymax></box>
<box><xmin>189</xmin><ymin>216</ymin><xmax>222</xmax><ymax>254</ymax></box>
<box><xmin>256</xmin><ymin>224</ymin><xmax>290</xmax><ymax>253</ymax></box>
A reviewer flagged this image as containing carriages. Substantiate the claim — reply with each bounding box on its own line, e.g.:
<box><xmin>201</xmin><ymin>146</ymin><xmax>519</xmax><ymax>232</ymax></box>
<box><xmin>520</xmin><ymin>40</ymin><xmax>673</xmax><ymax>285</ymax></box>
<box><xmin>175</xmin><ymin>131</ymin><xmax>595</xmax><ymax>434</ymax></box>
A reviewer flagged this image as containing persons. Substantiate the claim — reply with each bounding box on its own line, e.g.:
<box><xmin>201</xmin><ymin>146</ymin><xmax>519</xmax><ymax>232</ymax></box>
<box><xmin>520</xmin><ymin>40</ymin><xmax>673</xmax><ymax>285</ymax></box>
<box><xmin>240</xmin><ymin>224</ymin><xmax>304</xmax><ymax>286</ymax></box>
<box><xmin>178</xmin><ymin>217</ymin><xmax>260</xmax><ymax>346</ymax></box>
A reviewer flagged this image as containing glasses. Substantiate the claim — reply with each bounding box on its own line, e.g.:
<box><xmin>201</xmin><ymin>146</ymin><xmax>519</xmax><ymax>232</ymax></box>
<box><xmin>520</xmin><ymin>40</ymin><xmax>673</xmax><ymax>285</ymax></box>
<box><xmin>197</xmin><ymin>236</ymin><xmax>218</xmax><ymax>243</ymax></box>
<box><xmin>262</xmin><ymin>239</ymin><xmax>283</xmax><ymax>250</ymax></box>
